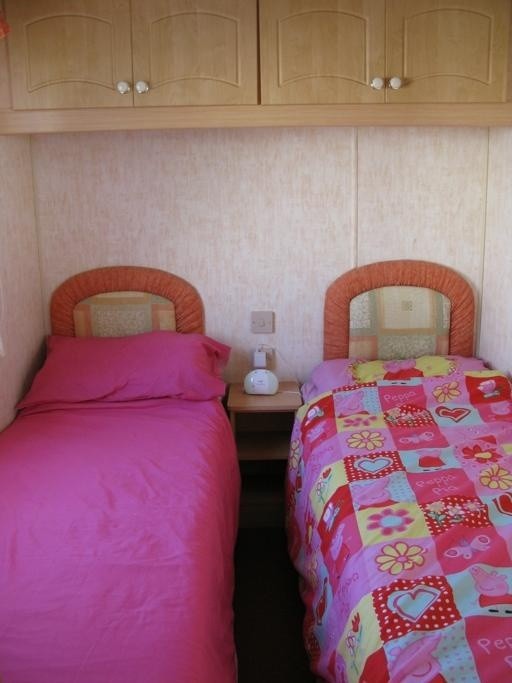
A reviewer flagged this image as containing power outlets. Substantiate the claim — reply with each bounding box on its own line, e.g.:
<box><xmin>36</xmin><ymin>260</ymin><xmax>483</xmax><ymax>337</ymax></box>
<box><xmin>257</xmin><ymin>348</ymin><xmax>275</xmax><ymax>371</ymax></box>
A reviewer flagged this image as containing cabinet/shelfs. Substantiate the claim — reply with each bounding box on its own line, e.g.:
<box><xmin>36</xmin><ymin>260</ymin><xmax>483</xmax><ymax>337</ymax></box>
<box><xmin>257</xmin><ymin>2</ymin><xmax>509</xmax><ymax>128</ymax></box>
<box><xmin>2</xmin><ymin>4</ymin><xmax>259</xmax><ymax>111</ymax></box>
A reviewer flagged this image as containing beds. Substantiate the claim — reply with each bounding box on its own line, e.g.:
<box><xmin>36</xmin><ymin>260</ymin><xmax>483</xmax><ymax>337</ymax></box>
<box><xmin>301</xmin><ymin>259</ymin><xmax>512</xmax><ymax>683</ymax></box>
<box><xmin>0</xmin><ymin>267</ymin><xmax>234</xmax><ymax>683</ymax></box>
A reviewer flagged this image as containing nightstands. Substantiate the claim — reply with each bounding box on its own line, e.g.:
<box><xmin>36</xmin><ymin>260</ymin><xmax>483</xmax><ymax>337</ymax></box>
<box><xmin>226</xmin><ymin>380</ymin><xmax>302</xmax><ymax>461</ymax></box>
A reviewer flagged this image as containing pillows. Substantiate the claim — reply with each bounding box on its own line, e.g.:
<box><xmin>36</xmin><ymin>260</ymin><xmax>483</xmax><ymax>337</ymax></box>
<box><xmin>13</xmin><ymin>332</ymin><xmax>232</xmax><ymax>410</ymax></box>
<box><xmin>311</xmin><ymin>353</ymin><xmax>489</xmax><ymax>394</ymax></box>
